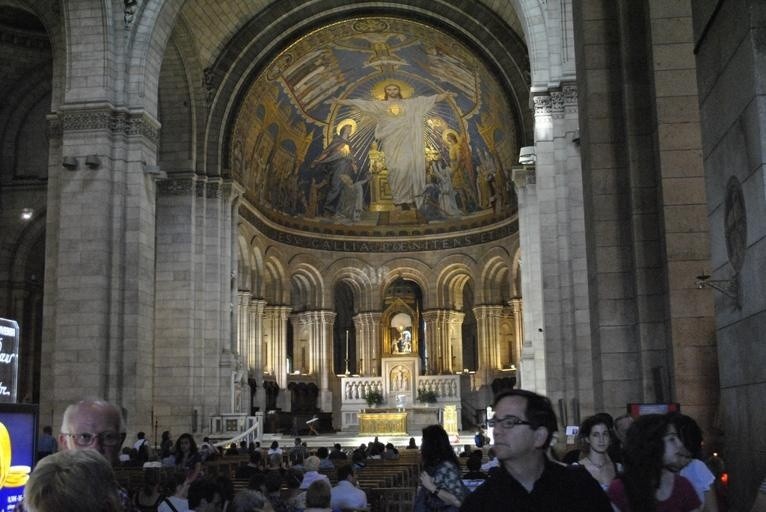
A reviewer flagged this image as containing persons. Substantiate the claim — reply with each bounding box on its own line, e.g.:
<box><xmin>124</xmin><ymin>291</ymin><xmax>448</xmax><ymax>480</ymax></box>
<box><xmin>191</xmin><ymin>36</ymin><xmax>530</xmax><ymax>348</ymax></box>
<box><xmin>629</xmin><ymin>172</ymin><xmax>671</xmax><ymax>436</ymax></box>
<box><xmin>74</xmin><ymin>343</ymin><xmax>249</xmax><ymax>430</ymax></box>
<box><xmin>13</xmin><ymin>397</ymin><xmax>401</xmax><ymax>511</ymax></box>
<box><xmin>407</xmin><ymin>389</ymin><xmax>766</xmax><ymax>511</ymax></box>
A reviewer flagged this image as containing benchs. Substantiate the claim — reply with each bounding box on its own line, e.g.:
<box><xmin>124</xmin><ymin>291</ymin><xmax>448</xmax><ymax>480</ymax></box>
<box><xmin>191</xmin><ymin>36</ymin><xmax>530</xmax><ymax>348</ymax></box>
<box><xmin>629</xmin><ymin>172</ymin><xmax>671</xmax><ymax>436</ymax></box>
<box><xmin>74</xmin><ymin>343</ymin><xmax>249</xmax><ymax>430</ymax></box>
<box><xmin>112</xmin><ymin>446</ymin><xmax>512</xmax><ymax>511</ymax></box>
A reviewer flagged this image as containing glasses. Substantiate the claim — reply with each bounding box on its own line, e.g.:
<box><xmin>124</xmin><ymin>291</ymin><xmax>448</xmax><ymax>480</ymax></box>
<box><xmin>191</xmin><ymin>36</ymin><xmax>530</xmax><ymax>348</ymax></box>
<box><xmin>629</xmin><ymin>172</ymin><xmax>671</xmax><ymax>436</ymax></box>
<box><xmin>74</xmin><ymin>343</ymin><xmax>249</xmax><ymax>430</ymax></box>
<box><xmin>487</xmin><ymin>417</ymin><xmax>530</xmax><ymax>429</ymax></box>
<box><xmin>63</xmin><ymin>432</ymin><xmax>121</xmax><ymax>446</ymax></box>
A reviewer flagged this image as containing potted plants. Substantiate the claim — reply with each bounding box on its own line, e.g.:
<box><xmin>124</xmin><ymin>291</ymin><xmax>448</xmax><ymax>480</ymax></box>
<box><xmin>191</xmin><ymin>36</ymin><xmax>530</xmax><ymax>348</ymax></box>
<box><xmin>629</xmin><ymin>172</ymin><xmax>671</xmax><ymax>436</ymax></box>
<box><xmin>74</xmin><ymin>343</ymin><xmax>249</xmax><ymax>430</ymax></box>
<box><xmin>361</xmin><ymin>388</ymin><xmax>384</xmax><ymax>408</ymax></box>
<box><xmin>416</xmin><ymin>388</ymin><xmax>439</xmax><ymax>408</ymax></box>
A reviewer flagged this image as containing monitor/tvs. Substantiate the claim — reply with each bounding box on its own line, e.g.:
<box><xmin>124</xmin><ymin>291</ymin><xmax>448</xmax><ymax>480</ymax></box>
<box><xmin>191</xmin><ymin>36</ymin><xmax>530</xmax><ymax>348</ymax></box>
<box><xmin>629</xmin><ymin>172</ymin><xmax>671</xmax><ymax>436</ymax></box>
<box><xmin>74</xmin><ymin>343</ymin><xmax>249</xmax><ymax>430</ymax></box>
<box><xmin>0</xmin><ymin>403</ymin><xmax>38</xmax><ymax>512</ymax></box>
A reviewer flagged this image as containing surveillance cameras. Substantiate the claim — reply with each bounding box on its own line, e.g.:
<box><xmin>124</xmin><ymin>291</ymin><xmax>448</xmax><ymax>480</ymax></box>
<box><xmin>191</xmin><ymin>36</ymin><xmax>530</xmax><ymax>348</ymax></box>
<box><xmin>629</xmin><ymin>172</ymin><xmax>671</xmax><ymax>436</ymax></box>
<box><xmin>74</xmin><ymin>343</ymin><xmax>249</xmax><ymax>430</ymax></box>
<box><xmin>571</xmin><ymin>133</ymin><xmax>581</xmax><ymax>143</ymax></box>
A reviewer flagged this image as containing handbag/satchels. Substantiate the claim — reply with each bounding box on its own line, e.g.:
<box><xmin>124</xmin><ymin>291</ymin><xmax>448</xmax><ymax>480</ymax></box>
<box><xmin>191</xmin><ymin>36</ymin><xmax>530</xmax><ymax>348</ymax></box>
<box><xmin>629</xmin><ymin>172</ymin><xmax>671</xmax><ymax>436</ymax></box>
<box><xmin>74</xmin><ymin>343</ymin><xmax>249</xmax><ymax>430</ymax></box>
<box><xmin>138</xmin><ymin>445</ymin><xmax>148</xmax><ymax>458</ymax></box>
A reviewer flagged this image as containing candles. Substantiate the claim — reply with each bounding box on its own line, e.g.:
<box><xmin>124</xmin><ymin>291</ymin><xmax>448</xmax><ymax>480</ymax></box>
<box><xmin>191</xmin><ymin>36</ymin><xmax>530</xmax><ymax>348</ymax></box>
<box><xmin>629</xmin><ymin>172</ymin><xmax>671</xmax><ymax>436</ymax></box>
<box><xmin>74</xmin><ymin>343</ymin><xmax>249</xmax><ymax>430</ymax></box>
<box><xmin>346</xmin><ymin>329</ymin><xmax>349</xmax><ymax>359</ymax></box>
<box><xmin>424</xmin><ymin>326</ymin><xmax>427</xmax><ymax>357</ymax></box>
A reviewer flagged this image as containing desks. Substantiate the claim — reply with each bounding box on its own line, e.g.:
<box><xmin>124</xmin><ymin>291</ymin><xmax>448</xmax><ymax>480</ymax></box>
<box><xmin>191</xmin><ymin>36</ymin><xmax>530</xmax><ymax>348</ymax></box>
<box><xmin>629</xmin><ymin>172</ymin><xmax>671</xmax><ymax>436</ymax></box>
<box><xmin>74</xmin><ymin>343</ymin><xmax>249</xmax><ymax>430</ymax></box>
<box><xmin>356</xmin><ymin>412</ymin><xmax>411</xmax><ymax>436</ymax></box>
